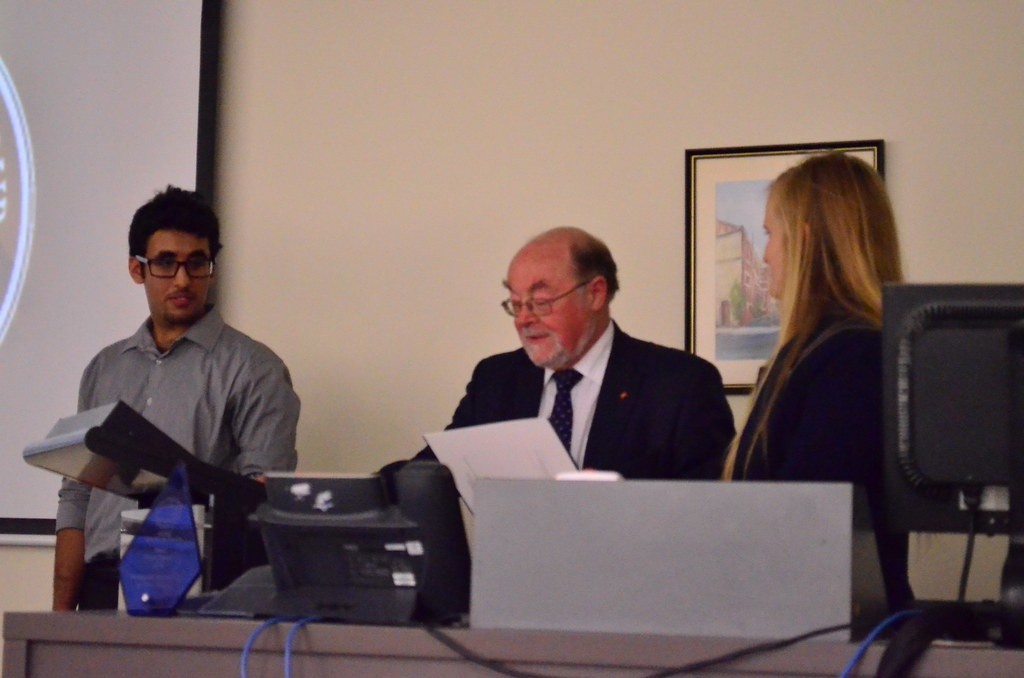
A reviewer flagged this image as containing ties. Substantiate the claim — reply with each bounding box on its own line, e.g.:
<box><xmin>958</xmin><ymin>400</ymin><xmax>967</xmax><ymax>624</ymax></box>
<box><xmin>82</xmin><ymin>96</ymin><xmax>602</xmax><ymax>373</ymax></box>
<box><xmin>549</xmin><ymin>370</ymin><xmax>583</xmax><ymax>451</ymax></box>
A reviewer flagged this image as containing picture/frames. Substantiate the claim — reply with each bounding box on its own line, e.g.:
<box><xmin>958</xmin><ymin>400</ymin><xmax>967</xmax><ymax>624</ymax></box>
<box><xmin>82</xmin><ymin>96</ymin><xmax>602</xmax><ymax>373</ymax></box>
<box><xmin>683</xmin><ymin>138</ymin><xmax>888</xmax><ymax>394</ymax></box>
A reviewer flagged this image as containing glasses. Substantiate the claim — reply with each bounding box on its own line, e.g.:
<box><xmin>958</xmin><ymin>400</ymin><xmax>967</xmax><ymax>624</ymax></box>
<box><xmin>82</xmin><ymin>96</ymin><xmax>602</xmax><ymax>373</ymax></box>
<box><xmin>136</xmin><ymin>254</ymin><xmax>214</xmax><ymax>279</ymax></box>
<box><xmin>502</xmin><ymin>281</ymin><xmax>590</xmax><ymax>318</ymax></box>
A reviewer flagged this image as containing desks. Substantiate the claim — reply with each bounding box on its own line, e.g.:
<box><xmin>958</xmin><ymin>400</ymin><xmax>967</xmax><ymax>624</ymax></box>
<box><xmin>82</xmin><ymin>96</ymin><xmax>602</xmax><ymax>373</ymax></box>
<box><xmin>1</xmin><ymin>603</ymin><xmax>1024</xmax><ymax>678</ymax></box>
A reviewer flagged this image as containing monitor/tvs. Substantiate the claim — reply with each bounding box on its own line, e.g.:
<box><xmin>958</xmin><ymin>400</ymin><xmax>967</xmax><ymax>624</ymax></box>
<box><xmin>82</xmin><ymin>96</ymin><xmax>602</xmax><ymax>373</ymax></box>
<box><xmin>874</xmin><ymin>283</ymin><xmax>1024</xmax><ymax>647</ymax></box>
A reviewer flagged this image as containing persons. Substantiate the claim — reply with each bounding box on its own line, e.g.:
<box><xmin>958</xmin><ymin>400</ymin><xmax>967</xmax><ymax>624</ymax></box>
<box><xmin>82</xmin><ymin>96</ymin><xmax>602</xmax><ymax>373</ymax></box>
<box><xmin>53</xmin><ymin>189</ymin><xmax>299</xmax><ymax>613</ymax></box>
<box><xmin>377</xmin><ymin>229</ymin><xmax>736</xmax><ymax>480</ymax></box>
<box><xmin>724</xmin><ymin>153</ymin><xmax>904</xmax><ymax>483</ymax></box>
